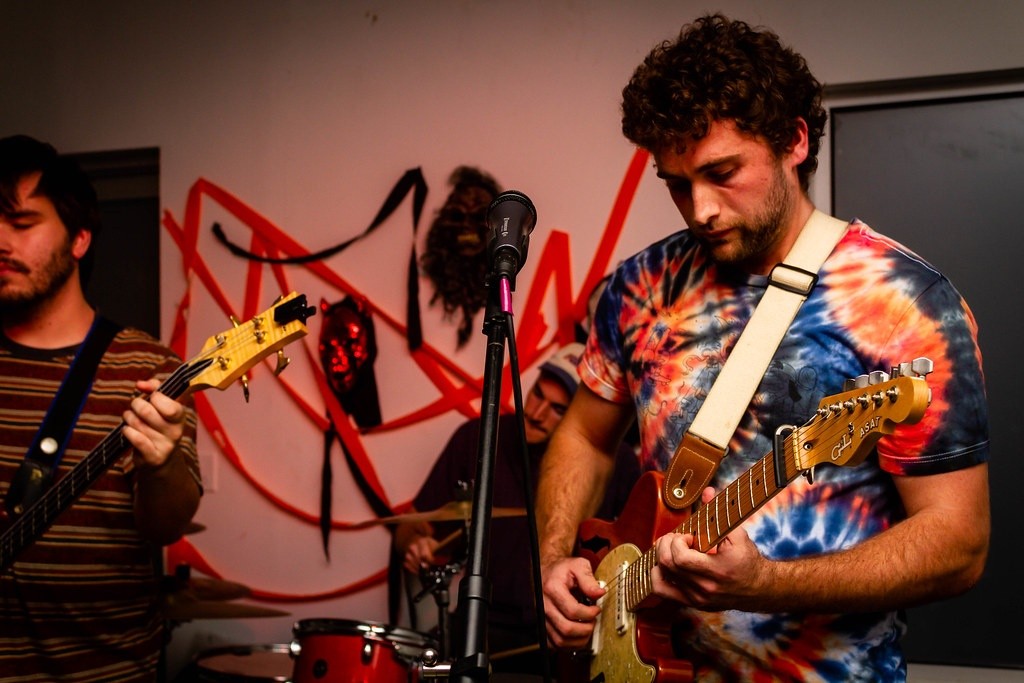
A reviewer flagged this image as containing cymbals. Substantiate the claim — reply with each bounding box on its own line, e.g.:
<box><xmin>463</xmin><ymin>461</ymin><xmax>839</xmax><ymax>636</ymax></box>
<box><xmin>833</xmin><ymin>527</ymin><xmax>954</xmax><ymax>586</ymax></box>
<box><xmin>375</xmin><ymin>500</ymin><xmax>531</xmax><ymax>524</ymax></box>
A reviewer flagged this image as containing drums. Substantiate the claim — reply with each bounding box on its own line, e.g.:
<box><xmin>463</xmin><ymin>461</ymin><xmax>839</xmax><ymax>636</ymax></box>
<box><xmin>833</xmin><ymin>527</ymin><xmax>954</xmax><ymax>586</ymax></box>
<box><xmin>290</xmin><ymin>614</ymin><xmax>449</xmax><ymax>683</ymax></box>
<box><xmin>194</xmin><ymin>640</ymin><xmax>294</xmax><ymax>682</ymax></box>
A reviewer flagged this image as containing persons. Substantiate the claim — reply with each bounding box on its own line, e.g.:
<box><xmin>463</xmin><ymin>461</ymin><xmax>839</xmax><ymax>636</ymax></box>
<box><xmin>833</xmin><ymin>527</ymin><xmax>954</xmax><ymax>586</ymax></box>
<box><xmin>535</xmin><ymin>12</ymin><xmax>991</xmax><ymax>683</ymax></box>
<box><xmin>0</xmin><ymin>135</ymin><xmax>205</xmax><ymax>683</ymax></box>
<box><xmin>395</xmin><ymin>342</ymin><xmax>585</xmax><ymax>683</ymax></box>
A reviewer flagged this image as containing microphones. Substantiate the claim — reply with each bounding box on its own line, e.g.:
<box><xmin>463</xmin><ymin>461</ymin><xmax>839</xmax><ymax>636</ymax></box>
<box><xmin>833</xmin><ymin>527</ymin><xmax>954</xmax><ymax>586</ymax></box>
<box><xmin>482</xmin><ymin>189</ymin><xmax>538</xmax><ymax>280</ymax></box>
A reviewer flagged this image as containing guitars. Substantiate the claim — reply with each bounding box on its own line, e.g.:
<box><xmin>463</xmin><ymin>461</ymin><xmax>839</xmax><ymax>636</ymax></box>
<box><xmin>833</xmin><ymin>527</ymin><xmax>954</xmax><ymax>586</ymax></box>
<box><xmin>0</xmin><ymin>291</ymin><xmax>319</xmax><ymax>577</ymax></box>
<box><xmin>560</xmin><ymin>356</ymin><xmax>933</xmax><ymax>683</ymax></box>
